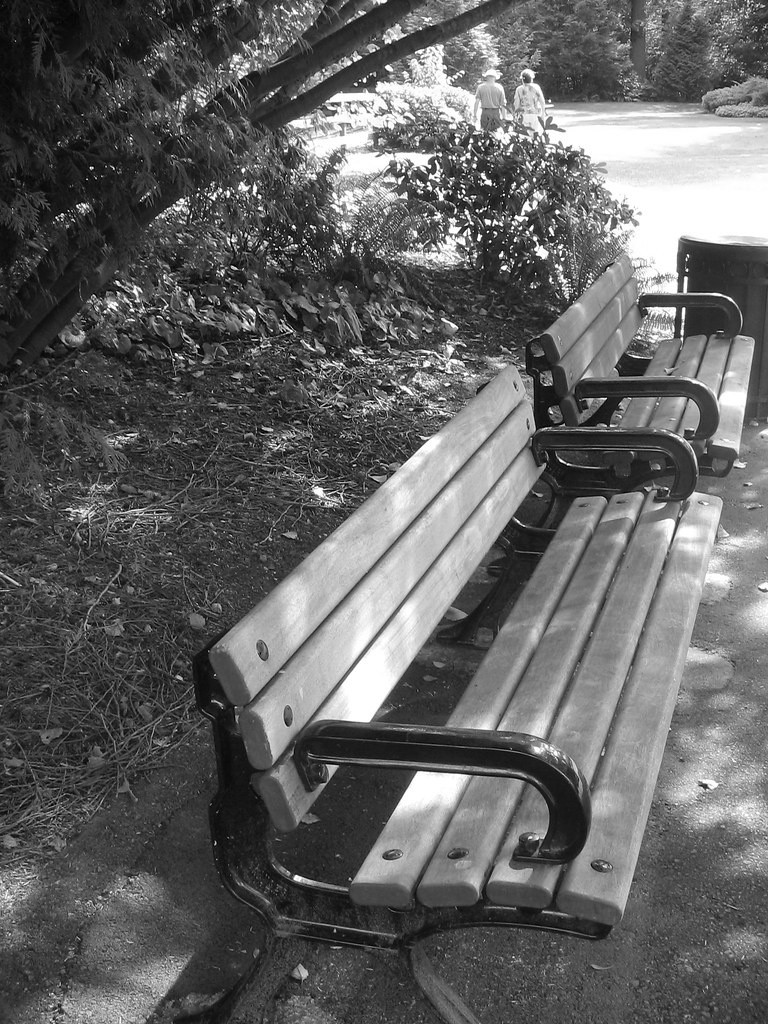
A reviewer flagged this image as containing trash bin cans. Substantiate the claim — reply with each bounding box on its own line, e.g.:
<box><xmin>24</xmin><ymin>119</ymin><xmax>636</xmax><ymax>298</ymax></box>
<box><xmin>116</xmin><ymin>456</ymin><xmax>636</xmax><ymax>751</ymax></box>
<box><xmin>674</xmin><ymin>235</ymin><xmax>768</xmax><ymax>421</ymax></box>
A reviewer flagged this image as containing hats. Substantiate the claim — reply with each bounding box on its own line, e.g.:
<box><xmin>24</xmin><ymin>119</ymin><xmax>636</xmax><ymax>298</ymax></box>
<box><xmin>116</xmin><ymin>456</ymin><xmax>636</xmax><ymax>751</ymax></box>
<box><xmin>481</xmin><ymin>69</ymin><xmax>501</xmax><ymax>80</ymax></box>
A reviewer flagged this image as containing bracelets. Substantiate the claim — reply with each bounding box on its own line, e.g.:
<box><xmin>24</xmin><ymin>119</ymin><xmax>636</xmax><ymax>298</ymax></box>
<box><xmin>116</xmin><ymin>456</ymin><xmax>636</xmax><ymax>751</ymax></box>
<box><xmin>473</xmin><ymin>116</ymin><xmax>476</xmax><ymax>117</ymax></box>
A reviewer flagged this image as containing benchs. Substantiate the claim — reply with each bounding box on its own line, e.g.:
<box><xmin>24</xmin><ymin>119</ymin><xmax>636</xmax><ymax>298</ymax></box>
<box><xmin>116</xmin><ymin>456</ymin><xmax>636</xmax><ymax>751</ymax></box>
<box><xmin>525</xmin><ymin>256</ymin><xmax>755</xmax><ymax>494</ymax></box>
<box><xmin>189</xmin><ymin>359</ymin><xmax>723</xmax><ymax>1024</ymax></box>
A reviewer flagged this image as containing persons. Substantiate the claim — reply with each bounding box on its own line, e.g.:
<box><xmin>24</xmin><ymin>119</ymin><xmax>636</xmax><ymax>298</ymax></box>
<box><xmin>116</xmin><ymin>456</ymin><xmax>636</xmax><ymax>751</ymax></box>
<box><xmin>472</xmin><ymin>70</ymin><xmax>506</xmax><ymax>134</ymax></box>
<box><xmin>514</xmin><ymin>69</ymin><xmax>545</xmax><ymax>137</ymax></box>
